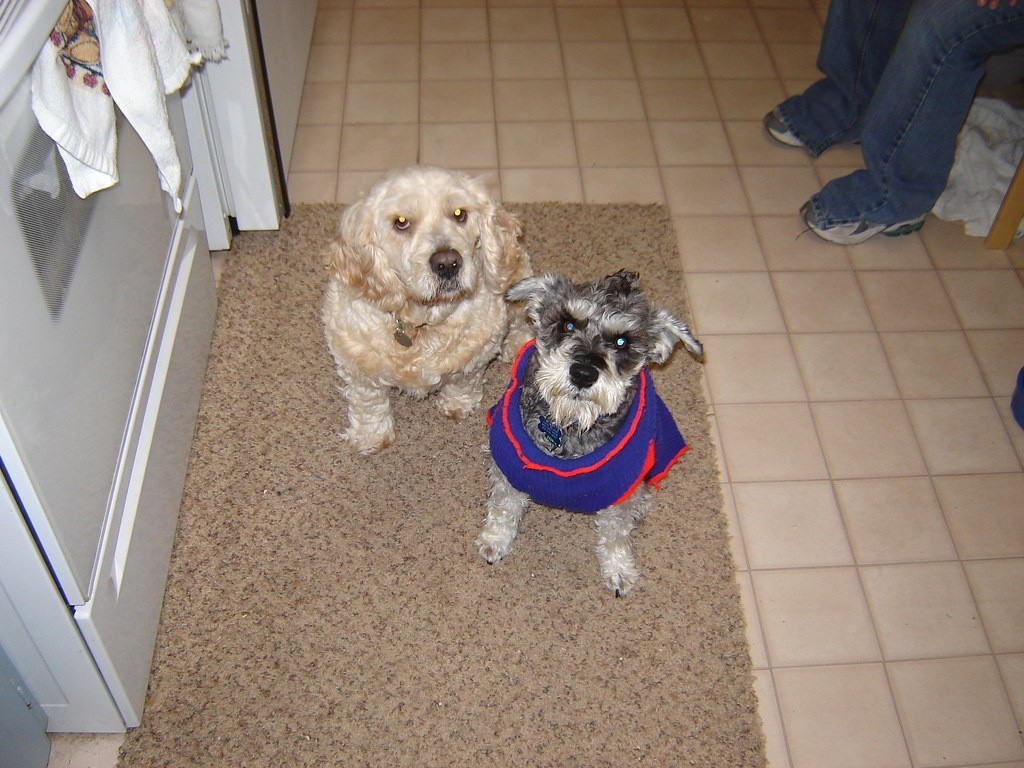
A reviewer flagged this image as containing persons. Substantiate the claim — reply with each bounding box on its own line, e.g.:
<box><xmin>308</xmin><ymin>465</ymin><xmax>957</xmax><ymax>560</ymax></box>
<box><xmin>764</xmin><ymin>0</ymin><xmax>1024</xmax><ymax>248</ymax></box>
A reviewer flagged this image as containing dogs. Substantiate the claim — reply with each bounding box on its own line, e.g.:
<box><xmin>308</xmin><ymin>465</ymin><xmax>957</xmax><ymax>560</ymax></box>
<box><xmin>322</xmin><ymin>165</ymin><xmax>708</xmax><ymax>599</ymax></box>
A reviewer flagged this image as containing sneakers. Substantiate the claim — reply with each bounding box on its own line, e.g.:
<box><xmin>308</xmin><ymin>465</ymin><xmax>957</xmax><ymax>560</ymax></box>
<box><xmin>800</xmin><ymin>200</ymin><xmax>926</xmax><ymax>245</ymax></box>
<box><xmin>764</xmin><ymin>107</ymin><xmax>863</xmax><ymax>146</ymax></box>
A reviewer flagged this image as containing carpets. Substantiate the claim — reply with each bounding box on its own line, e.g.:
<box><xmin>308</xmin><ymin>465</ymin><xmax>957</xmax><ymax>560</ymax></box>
<box><xmin>118</xmin><ymin>201</ymin><xmax>766</xmax><ymax>767</ymax></box>
